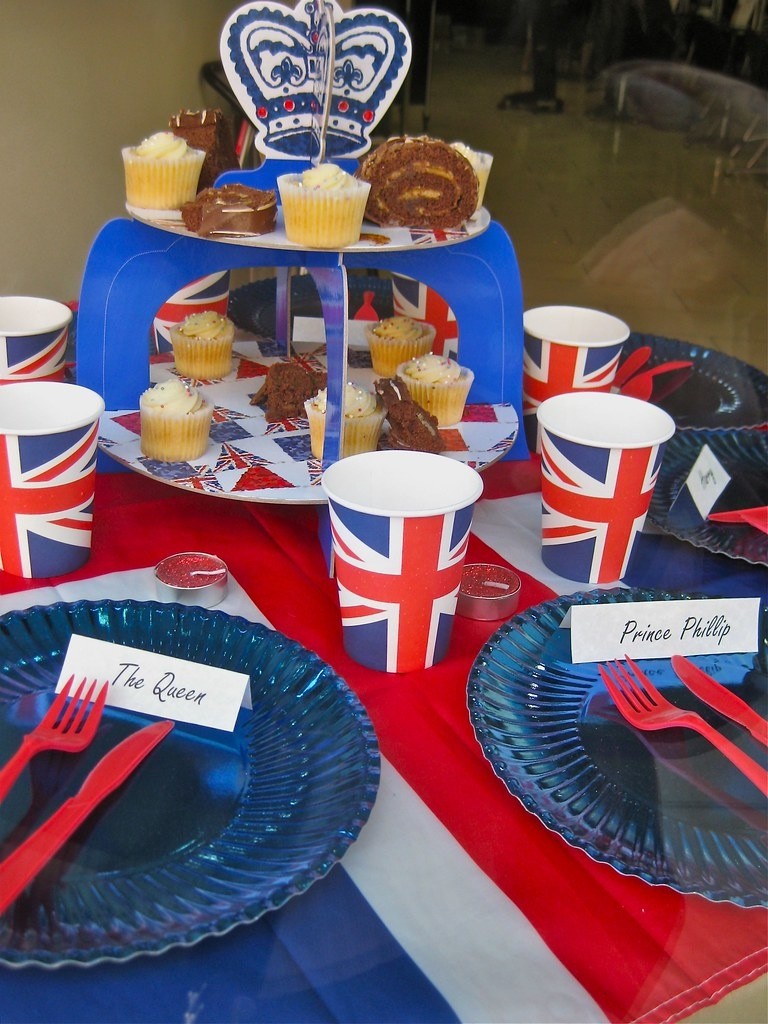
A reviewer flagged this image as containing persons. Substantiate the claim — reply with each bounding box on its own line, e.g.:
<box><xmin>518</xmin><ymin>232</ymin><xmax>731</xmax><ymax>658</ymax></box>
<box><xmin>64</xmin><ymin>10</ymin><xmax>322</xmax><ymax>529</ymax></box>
<box><xmin>452</xmin><ymin>0</ymin><xmax>768</xmax><ymax>114</ymax></box>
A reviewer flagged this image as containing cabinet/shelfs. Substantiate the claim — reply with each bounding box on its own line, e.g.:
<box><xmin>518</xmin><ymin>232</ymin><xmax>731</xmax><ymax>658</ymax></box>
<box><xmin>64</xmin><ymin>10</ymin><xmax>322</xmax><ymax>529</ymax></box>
<box><xmin>74</xmin><ymin>202</ymin><xmax>531</xmax><ymax>578</ymax></box>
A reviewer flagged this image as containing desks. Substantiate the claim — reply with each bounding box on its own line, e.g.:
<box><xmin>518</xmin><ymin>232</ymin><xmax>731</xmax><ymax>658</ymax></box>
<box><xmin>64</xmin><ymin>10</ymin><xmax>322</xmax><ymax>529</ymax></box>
<box><xmin>0</xmin><ymin>301</ymin><xmax>768</xmax><ymax>1024</ymax></box>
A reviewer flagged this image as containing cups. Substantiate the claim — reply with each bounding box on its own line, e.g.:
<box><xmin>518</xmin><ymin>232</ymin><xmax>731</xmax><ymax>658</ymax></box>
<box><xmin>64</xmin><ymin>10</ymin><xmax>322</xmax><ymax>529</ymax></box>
<box><xmin>535</xmin><ymin>389</ymin><xmax>675</xmax><ymax>583</ymax></box>
<box><xmin>0</xmin><ymin>381</ymin><xmax>103</xmax><ymax>578</ymax></box>
<box><xmin>518</xmin><ymin>305</ymin><xmax>632</xmax><ymax>452</ymax></box>
<box><xmin>391</xmin><ymin>270</ymin><xmax>458</xmax><ymax>363</ymax></box>
<box><xmin>321</xmin><ymin>449</ymin><xmax>484</xmax><ymax>674</ymax></box>
<box><xmin>1</xmin><ymin>295</ymin><xmax>72</xmax><ymax>392</ymax></box>
<box><xmin>150</xmin><ymin>268</ymin><xmax>231</xmax><ymax>355</ymax></box>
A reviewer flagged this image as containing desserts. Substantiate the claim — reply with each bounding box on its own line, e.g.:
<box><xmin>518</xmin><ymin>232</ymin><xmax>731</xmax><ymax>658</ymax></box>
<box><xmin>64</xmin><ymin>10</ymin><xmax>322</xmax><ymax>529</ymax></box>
<box><xmin>122</xmin><ymin>106</ymin><xmax>493</xmax><ymax>460</ymax></box>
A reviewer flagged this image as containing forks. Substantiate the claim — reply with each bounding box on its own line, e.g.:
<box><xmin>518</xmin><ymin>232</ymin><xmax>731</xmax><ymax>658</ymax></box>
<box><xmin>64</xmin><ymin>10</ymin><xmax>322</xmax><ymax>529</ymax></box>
<box><xmin>598</xmin><ymin>655</ymin><xmax>767</xmax><ymax>809</ymax></box>
<box><xmin>0</xmin><ymin>673</ymin><xmax>110</xmax><ymax>804</ymax></box>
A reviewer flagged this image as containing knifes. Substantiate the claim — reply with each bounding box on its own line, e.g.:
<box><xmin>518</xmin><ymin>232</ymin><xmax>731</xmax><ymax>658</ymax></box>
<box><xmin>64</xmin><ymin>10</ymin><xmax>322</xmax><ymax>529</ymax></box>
<box><xmin>670</xmin><ymin>655</ymin><xmax>767</xmax><ymax>751</ymax></box>
<box><xmin>0</xmin><ymin>719</ymin><xmax>176</xmax><ymax>923</ymax></box>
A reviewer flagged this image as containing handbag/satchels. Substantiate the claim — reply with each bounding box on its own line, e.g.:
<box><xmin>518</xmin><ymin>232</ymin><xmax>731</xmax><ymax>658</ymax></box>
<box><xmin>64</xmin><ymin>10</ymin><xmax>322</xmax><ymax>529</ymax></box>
<box><xmin>201</xmin><ymin>60</ymin><xmax>264</xmax><ymax>172</ymax></box>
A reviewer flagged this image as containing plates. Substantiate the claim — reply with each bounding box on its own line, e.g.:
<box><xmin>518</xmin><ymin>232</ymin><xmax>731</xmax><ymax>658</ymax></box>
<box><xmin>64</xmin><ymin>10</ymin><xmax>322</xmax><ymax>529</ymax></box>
<box><xmin>229</xmin><ymin>274</ymin><xmax>394</xmax><ymax>344</ymax></box>
<box><xmin>465</xmin><ymin>585</ymin><xmax>767</xmax><ymax>914</ymax></box>
<box><xmin>1</xmin><ymin>597</ymin><xmax>380</xmax><ymax>974</ymax></box>
